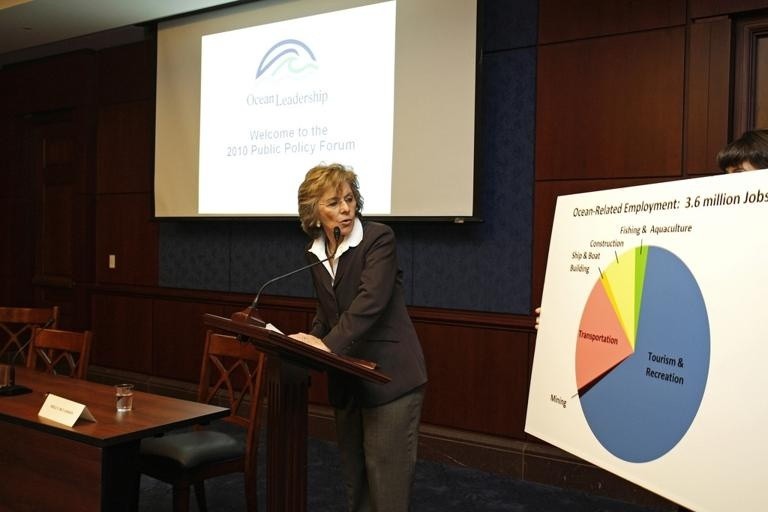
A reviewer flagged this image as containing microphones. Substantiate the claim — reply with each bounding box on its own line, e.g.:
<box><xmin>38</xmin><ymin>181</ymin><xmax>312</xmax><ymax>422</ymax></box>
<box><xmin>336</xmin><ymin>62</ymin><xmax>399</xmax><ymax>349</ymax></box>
<box><xmin>0</xmin><ymin>317</ymin><xmax>56</xmax><ymax>396</ymax></box>
<box><xmin>231</xmin><ymin>226</ymin><xmax>340</xmax><ymax>327</ymax></box>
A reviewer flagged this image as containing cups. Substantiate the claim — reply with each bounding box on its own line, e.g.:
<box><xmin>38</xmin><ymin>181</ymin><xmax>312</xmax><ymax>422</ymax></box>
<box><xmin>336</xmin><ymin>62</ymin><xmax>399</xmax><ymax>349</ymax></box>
<box><xmin>115</xmin><ymin>383</ymin><xmax>135</xmax><ymax>412</ymax></box>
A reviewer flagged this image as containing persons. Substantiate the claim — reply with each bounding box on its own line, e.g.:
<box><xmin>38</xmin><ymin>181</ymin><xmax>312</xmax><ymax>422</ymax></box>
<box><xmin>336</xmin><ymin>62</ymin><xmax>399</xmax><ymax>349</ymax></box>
<box><xmin>287</xmin><ymin>164</ymin><xmax>428</xmax><ymax>512</ymax></box>
<box><xmin>535</xmin><ymin>128</ymin><xmax>768</xmax><ymax>330</ymax></box>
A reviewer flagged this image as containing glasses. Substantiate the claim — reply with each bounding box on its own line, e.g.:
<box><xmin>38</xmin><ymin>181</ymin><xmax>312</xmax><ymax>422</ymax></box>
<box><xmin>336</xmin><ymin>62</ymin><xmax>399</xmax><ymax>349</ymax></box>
<box><xmin>324</xmin><ymin>192</ymin><xmax>354</xmax><ymax>208</ymax></box>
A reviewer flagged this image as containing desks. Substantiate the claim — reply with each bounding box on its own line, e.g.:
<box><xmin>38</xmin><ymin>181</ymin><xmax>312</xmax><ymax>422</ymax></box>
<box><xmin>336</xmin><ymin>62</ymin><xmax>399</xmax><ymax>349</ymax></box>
<box><xmin>0</xmin><ymin>363</ymin><xmax>231</xmax><ymax>511</ymax></box>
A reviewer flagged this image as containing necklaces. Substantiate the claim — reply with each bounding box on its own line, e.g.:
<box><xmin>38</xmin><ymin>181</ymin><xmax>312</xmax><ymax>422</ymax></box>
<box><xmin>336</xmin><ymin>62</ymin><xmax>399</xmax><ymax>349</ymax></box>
<box><xmin>324</xmin><ymin>242</ymin><xmax>335</xmax><ymax>258</ymax></box>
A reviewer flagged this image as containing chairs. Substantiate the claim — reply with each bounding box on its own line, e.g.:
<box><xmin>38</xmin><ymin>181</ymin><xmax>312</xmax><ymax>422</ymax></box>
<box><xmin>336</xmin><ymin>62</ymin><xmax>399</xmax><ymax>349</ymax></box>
<box><xmin>140</xmin><ymin>329</ymin><xmax>267</xmax><ymax>510</ymax></box>
<box><xmin>0</xmin><ymin>304</ymin><xmax>94</xmax><ymax>381</ymax></box>
